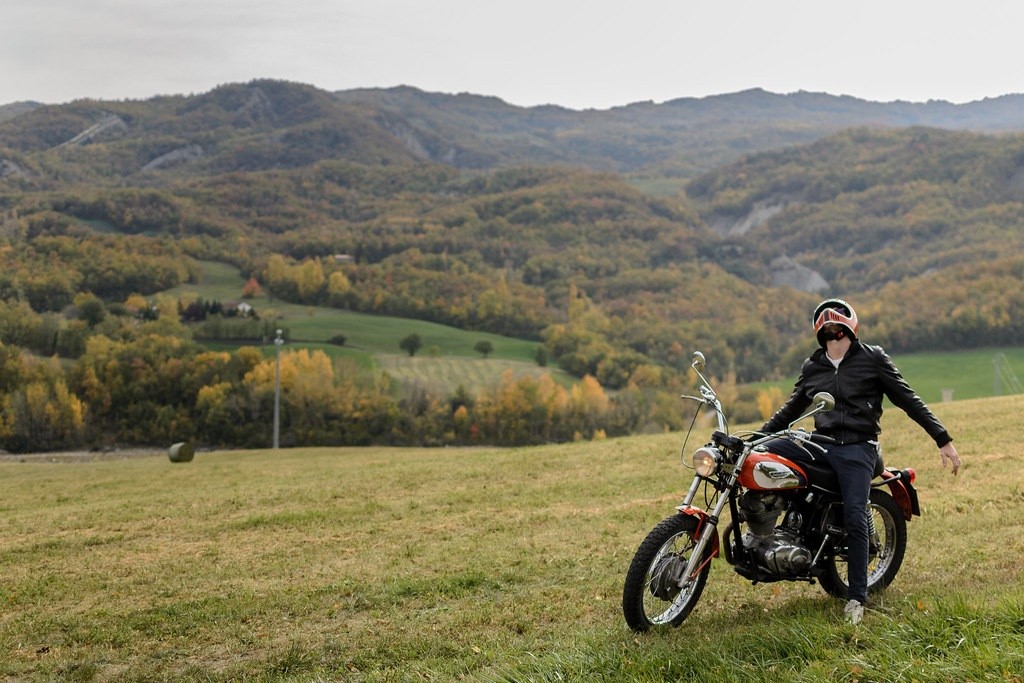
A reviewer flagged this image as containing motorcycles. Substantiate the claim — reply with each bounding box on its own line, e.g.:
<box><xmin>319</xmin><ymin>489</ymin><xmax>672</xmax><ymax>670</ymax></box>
<box><xmin>620</xmin><ymin>350</ymin><xmax>922</xmax><ymax>636</ymax></box>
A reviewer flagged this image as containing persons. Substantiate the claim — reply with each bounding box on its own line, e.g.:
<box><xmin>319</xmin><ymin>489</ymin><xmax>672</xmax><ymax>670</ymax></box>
<box><xmin>740</xmin><ymin>297</ymin><xmax>963</xmax><ymax>628</ymax></box>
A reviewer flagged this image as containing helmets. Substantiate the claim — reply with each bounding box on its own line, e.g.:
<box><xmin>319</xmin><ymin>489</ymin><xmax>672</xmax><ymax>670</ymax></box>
<box><xmin>811</xmin><ymin>298</ymin><xmax>859</xmax><ymax>348</ymax></box>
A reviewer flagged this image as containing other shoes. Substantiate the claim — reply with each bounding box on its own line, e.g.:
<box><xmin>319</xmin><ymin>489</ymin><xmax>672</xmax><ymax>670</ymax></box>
<box><xmin>843</xmin><ymin>599</ymin><xmax>863</xmax><ymax>623</ymax></box>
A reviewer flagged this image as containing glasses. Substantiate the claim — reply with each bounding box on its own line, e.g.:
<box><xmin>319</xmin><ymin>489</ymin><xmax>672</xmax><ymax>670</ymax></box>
<box><xmin>835</xmin><ymin>308</ymin><xmax>846</xmax><ymax>314</ymax></box>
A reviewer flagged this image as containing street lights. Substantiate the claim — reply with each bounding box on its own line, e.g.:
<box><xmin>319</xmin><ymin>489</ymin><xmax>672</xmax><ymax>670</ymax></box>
<box><xmin>272</xmin><ymin>328</ymin><xmax>286</xmax><ymax>454</ymax></box>
